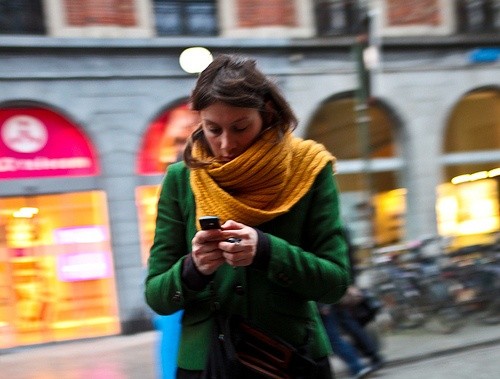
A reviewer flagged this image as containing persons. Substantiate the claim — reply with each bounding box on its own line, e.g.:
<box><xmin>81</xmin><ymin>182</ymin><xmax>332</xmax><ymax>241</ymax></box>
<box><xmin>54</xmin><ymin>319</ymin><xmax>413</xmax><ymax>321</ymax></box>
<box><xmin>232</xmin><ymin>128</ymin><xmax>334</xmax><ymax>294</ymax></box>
<box><xmin>143</xmin><ymin>55</ymin><xmax>353</xmax><ymax>378</ymax></box>
<box><xmin>147</xmin><ymin>305</ymin><xmax>187</xmax><ymax>378</ymax></box>
<box><xmin>321</xmin><ymin>280</ymin><xmax>389</xmax><ymax>378</ymax></box>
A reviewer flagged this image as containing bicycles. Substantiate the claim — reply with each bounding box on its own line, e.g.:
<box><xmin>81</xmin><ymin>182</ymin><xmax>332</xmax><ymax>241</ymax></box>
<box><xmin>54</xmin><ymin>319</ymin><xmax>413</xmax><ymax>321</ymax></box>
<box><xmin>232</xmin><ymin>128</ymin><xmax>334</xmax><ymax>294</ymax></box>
<box><xmin>350</xmin><ymin>229</ymin><xmax>499</xmax><ymax>331</ymax></box>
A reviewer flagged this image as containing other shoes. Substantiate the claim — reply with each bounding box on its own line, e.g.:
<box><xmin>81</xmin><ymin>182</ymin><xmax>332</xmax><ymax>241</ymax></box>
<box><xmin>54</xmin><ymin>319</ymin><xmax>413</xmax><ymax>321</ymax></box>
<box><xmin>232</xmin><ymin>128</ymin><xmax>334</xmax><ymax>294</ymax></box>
<box><xmin>353</xmin><ymin>352</ymin><xmax>383</xmax><ymax>379</ymax></box>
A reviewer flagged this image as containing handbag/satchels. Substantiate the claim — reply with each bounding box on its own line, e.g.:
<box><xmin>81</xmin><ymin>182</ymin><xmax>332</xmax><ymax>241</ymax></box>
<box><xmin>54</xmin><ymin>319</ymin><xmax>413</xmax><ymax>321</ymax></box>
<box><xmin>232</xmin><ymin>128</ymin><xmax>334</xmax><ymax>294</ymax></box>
<box><xmin>205</xmin><ymin>310</ymin><xmax>320</xmax><ymax>379</ymax></box>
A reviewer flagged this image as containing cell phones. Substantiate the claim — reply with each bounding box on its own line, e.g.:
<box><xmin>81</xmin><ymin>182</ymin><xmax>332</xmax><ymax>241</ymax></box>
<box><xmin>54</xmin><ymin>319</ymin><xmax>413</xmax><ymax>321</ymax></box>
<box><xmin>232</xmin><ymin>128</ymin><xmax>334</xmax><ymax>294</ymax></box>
<box><xmin>200</xmin><ymin>217</ymin><xmax>222</xmax><ymax>232</ymax></box>
